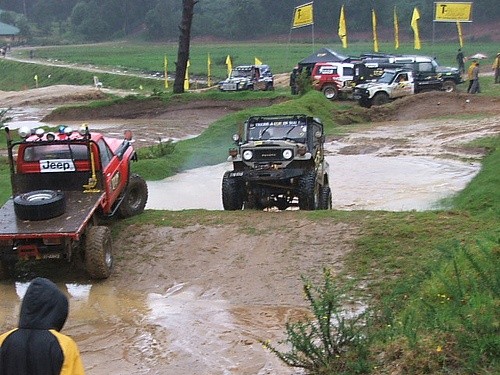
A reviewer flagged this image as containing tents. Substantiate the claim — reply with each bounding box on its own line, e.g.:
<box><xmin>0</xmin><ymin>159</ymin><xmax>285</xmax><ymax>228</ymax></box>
<box><xmin>298</xmin><ymin>46</ymin><xmax>351</xmax><ymax>68</ymax></box>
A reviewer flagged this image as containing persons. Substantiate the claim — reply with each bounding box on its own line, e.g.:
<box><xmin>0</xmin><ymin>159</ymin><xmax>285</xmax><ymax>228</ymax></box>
<box><xmin>397</xmin><ymin>75</ymin><xmax>404</xmax><ymax>82</ymax></box>
<box><xmin>290</xmin><ymin>67</ymin><xmax>312</xmax><ymax>96</ymax></box>
<box><xmin>0</xmin><ymin>276</ymin><xmax>85</xmax><ymax>375</ymax></box>
<box><xmin>0</xmin><ymin>43</ymin><xmax>11</xmax><ymax>57</ymax></box>
<box><xmin>456</xmin><ymin>48</ymin><xmax>500</xmax><ymax>94</ymax></box>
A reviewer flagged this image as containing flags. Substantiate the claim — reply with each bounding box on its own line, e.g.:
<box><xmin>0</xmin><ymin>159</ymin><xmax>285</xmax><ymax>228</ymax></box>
<box><xmin>255</xmin><ymin>57</ymin><xmax>261</xmax><ymax>65</ymax></box>
<box><xmin>183</xmin><ymin>60</ymin><xmax>191</xmax><ymax>90</ymax></box>
<box><xmin>411</xmin><ymin>8</ymin><xmax>422</xmax><ymax>49</ymax></box>
<box><xmin>165</xmin><ymin>55</ymin><xmax>169</xmax><ymax>89</ymax></box>
<box><xmin>370</xmin><ymin>10</ymin><xmax>378</xmax><ymax>53</ymax></box>
<box><xmin>393</xmin><ymin>8</ymin><xmax>399</xmax><ymax>49</ymax></box>
<box><xmin>207</xmin><ymin>56</ymin><xmax>211</xmax><ymax>87</ymax></box>
<box><xmin>226</xmin><ymin>55</ymin><xmax>233</xmax><ymax>74</ymax></box>
<box><xmin>336</xmin><ymin>7</ymin><xmax>347</xmax><ymax>49</ymax></box>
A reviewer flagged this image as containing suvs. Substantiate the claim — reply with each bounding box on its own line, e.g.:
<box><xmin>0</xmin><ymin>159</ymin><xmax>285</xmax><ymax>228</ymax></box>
<box><xmin>218</xmin><ymin>64</ymin><xmax>275</xmax><ymax>92</ymax></box>
<box><xmin>221</xmin><ymin>114</ymin><xmax>332</xmax><ymax>212</ymax></box>
<box><xmin>309</xmin><ymin>53</ymin><xmax>465</xmax><ymax>108</ymax></box>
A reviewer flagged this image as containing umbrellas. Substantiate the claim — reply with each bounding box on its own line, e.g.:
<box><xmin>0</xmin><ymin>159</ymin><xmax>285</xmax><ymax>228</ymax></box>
<box><xmin>471</xmin><ymin>53</ymin><xmax>488</xmax><ymax>59</ymax></box>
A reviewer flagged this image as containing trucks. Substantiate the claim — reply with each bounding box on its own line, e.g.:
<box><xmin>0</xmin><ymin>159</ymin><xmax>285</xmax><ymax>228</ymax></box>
<box><xmin>0</xmin><ymin>123</ymin><xmax>149</xmax><ymax>282</ymax></box>
